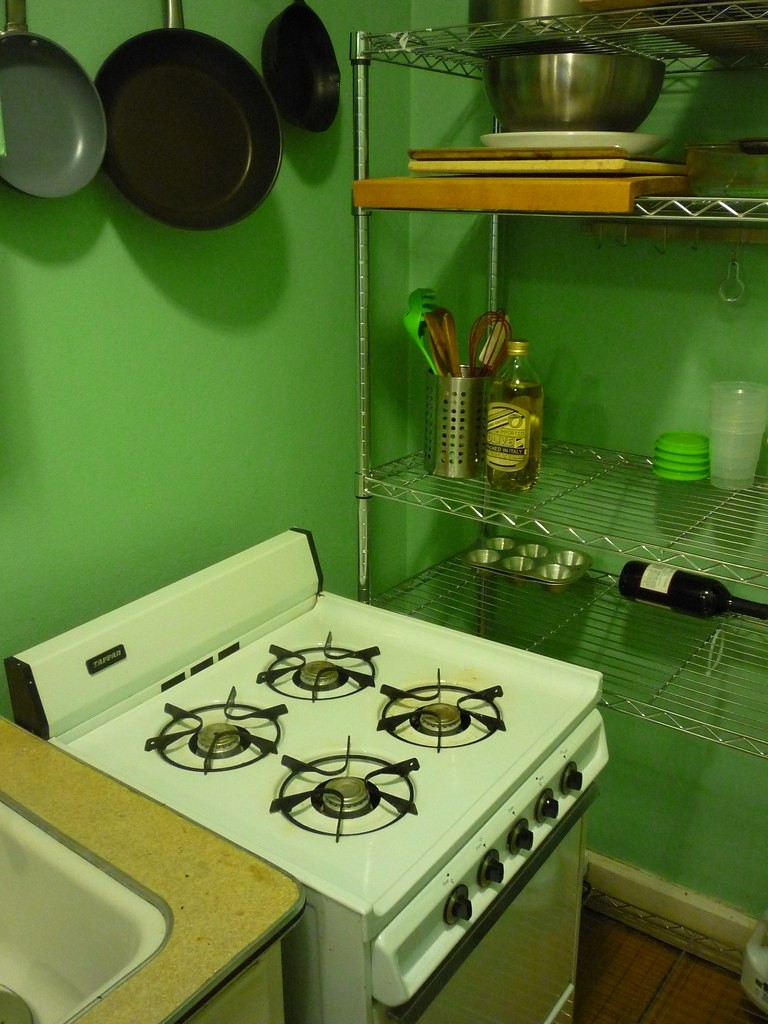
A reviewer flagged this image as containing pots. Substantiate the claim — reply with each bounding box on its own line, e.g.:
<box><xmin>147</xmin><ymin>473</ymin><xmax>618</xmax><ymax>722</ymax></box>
<box><xmin>0</xmin><ymin>0</ymin><xmax>106</xmax><ymax>199</ymax></box>
<box><xmin>473</xmin><ymin>53</ymin><xmax>679</xmax><ymax>131</ymax></box>
<box><xmin>92</xmin><ymin>0</ymin><xmax>282</xmax><ymax>231</ymax></box>
<box><xmin>261</xmin><ymin>0</ymin><xmax>342</xmax><ymax>133</ymax></box>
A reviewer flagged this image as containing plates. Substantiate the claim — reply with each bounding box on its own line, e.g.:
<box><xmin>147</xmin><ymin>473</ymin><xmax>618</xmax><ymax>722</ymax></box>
<box><xmin>480</xmin><ymin>133</ymin><xmax>675</xmax><ymax>155</ymax></box>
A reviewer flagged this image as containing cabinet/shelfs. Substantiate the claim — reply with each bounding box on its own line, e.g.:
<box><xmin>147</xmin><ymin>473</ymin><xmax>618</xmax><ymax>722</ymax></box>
<box><xmin>347</xmin><ymin>14</ymin><xmax>768</xmax><ymax>979</ymax></box>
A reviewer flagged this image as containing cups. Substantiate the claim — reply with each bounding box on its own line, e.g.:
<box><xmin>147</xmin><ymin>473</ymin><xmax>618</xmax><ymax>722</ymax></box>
<box><xmin>709</xmin><ymin>381</ymin><xmax>768</xmax><ymax>491</ymax></box>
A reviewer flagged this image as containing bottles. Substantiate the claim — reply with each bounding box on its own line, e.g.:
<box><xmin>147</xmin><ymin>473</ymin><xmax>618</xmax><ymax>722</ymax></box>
<box><xmin>618</xmin><ymin>561</ymin><xmax>768</xmax><ymax>620</ymax></box>
<box><xmin>485</xmin><ymin>339</ymin><xmax>544</xmax><ymax>493</ymax></box>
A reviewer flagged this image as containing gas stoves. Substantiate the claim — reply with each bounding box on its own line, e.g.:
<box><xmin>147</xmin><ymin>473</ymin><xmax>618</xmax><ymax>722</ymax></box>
<box><xmin>4</xmin><ymin>527</ymin><xmax>613</xmax><ymax>1006</ymax></box>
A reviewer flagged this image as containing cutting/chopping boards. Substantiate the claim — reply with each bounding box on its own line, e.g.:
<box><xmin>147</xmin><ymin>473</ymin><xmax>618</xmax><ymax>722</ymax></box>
<box><xmin>405</xmin><ymin>149</ymin><xmax>691</xmax><ymax>176</ymax></box>
<box><xmin>353</xmin><ymin>176</ymin><xmax>690</xmax><ymax>212</ymax></box>
